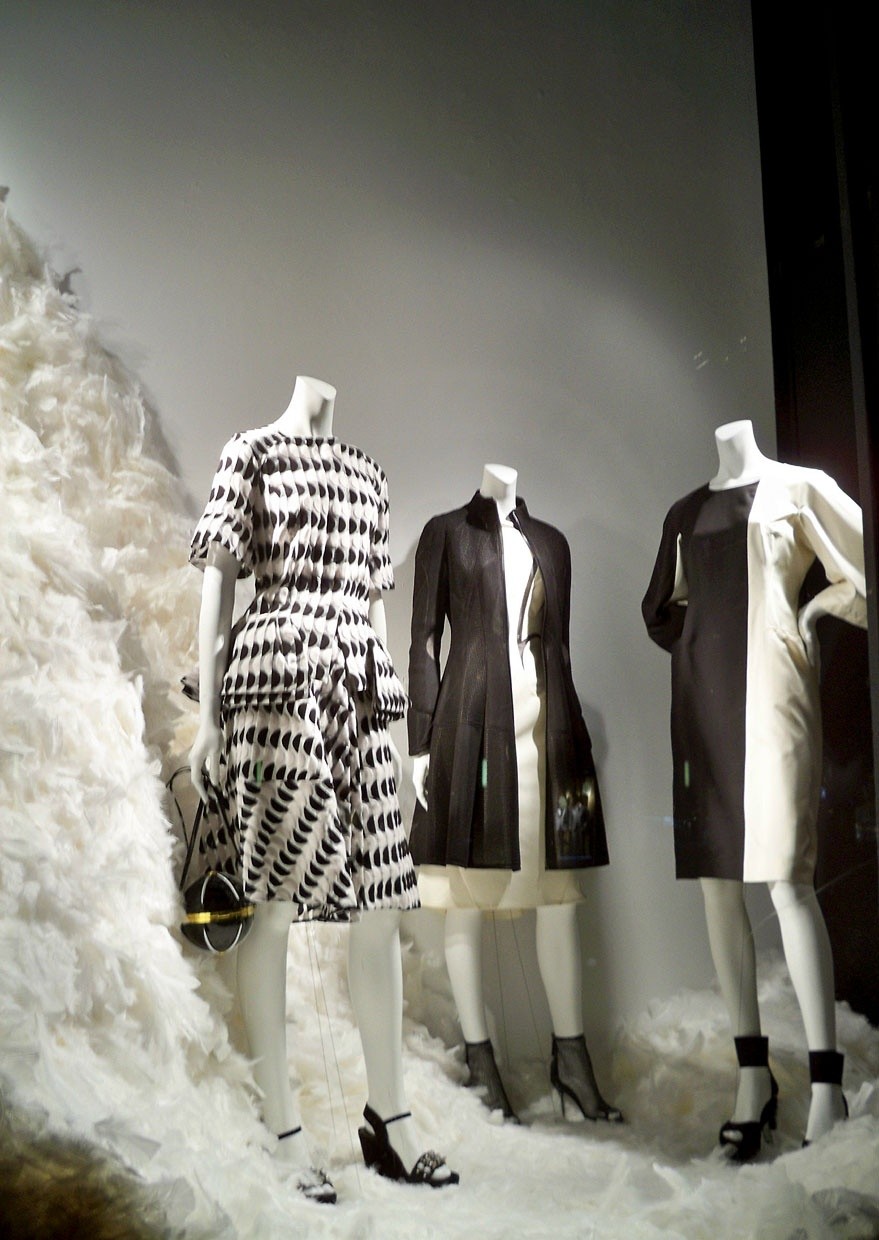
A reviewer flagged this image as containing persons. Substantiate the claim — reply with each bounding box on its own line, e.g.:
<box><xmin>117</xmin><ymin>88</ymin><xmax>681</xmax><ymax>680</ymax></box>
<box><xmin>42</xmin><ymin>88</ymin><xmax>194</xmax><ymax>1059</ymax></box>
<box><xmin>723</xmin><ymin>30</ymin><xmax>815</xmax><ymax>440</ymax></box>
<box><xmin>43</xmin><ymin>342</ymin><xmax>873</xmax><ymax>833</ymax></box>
<box><xmin>406</xmin><ymin>465</ymin><xmax>628</xmax><ymax>1129</ymax></box>
<box><xmin>187</xmin><ymin>375</ymin><xmax>462</xmax><ymax>1203</ymax></box>
<box><xmin>640</xmin><ymin>419</ymin><xmax>870</xmax><ymax>1166</ymax></box>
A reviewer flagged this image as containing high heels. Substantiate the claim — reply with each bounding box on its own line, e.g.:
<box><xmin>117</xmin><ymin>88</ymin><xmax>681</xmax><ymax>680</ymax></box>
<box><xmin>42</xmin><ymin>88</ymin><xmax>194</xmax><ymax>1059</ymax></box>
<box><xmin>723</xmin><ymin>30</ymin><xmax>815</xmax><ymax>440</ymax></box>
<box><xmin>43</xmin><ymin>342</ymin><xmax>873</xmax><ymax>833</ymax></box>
<box><xmin>548</xmin><ymin>1033</ymin><xmax>624</xmax><ymax>1122</ymax></box>
<box><xmin>719</xmin><ymin>1034</ymin><xmax>778</xmax><ymax>1162</ymax></box>
<box><xmin>358</xmin><ymin>1103</ymin><xmax>461</xmax><ymax>1189</ymax></box>
<box><xmin>802</xmin><ymin>1049</ymin><xmax>847</xmax><ymax>1149</ymax></box>
<box><xmin>275</xmin><ymin>1126</ymin><xmax>338</xmax><ymax>1204</ymax></box>
<box><xmin>466</xmin><ymin>1039</ymin><xmax>522</xmax><ymax>1125</ymax></box>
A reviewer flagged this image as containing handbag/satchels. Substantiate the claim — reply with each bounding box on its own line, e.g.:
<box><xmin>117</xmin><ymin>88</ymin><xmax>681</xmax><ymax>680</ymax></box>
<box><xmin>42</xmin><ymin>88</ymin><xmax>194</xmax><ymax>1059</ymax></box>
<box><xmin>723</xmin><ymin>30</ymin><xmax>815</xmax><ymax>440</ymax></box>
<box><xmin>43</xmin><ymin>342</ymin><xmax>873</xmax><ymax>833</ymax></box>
<box><xmin>164</xmin><ymin>767</ymin><xmax>254</xmax><ymax>955</ymax></box>
